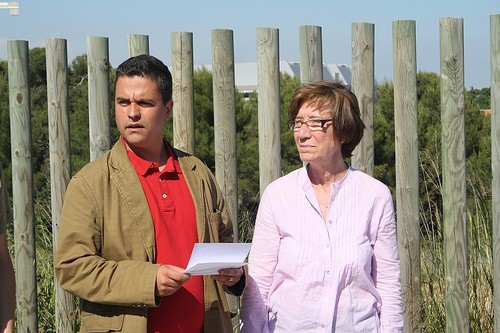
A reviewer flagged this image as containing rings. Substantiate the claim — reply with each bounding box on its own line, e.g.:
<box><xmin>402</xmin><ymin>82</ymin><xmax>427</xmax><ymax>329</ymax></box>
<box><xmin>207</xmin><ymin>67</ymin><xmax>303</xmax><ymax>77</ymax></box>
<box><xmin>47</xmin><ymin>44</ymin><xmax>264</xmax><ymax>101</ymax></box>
<box><xmin>229</xmin><ymin>276</ymin><xmax>233</xmax><ymax>281</ymax></box>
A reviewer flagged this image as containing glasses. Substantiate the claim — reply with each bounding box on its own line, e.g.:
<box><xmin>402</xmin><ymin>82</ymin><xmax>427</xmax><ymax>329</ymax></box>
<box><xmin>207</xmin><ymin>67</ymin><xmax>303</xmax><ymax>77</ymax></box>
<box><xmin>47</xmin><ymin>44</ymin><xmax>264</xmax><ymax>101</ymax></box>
<box><xmin>287</xmin><ymin>118</ymin><xmax>335</xmax><ymax>131</ymax></box>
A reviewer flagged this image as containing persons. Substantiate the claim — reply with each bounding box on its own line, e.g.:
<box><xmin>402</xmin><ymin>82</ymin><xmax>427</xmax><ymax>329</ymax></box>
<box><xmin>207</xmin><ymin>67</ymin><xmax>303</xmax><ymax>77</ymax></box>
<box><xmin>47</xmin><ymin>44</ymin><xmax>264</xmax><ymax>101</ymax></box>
<box><xmin>55</xmin><ymin>53</ymin><xmax>246</xmax><ymax>333</ymax></box>
<box><xmin>240</xmin><ymin>79</ymin><xmax>403</xmax><ymax>333</ymax></box>
<box><xmin>0</xmin><ymin>162</ymin><xmax>17</xmax><ymax>333</ymax></box>
